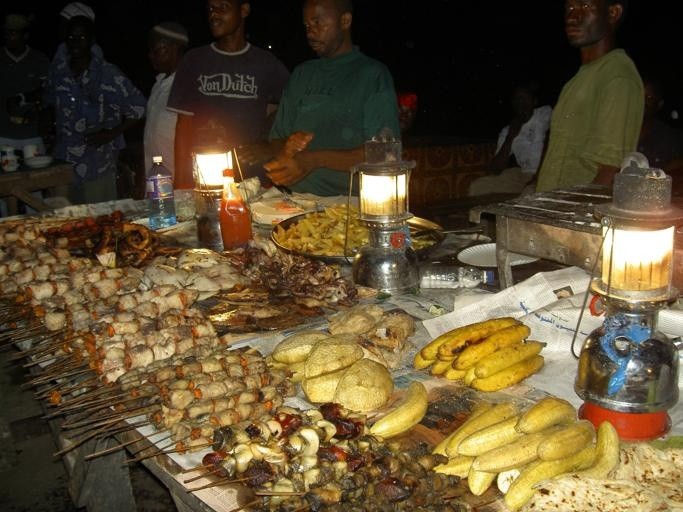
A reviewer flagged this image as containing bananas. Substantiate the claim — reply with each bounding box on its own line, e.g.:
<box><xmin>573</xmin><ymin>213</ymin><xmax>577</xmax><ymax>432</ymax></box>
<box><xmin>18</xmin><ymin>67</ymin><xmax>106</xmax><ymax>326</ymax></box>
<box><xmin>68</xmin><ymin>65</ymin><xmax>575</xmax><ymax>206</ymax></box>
<box><xmin>370</xmin><ymin>381</ymin><xmax>428</xmax><ymax>439</ymax></box>
<box><xmin>433</xmin><ymin>396</ymin><xmax>621</xmax><ymax>511</ymax></box>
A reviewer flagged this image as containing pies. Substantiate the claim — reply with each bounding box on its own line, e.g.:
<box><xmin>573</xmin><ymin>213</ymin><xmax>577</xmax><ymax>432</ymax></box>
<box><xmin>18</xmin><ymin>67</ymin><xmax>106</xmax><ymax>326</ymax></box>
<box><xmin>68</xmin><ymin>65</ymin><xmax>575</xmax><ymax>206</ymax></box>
<box><xmin>520</xmin><ymin>444</ymin><xmax>683</xmax><ymax>511</ymax></box>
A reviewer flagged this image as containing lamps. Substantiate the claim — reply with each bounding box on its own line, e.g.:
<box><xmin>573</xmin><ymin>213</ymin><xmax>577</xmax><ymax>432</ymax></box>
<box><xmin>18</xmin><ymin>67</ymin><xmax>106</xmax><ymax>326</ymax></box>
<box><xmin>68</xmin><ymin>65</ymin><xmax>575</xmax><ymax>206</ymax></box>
<box><xmin>187</xmin><ymin>117</ymin><xmax>236</xmax><ymax>250</ymax></box>
<box><xmin>343</xmin><ymin>125</ymin><xmax>421</xmax><ymax>294</ymax></box>
<box><xmin>571</xmin><ymin>149</ymin><xmax>683</xmax><ymax>444</ymax></box>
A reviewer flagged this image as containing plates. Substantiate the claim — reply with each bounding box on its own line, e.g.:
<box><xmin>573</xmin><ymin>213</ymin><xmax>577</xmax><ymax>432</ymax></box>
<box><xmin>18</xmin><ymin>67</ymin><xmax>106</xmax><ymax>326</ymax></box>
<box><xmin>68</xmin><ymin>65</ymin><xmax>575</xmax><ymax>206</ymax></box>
<box><xmin>23</xmin><ymin>156</ymin><xmax>53</xmax><ymax>169</ymax></box>
<box><xmin>250</xmin><ymin>197</ymin><xmax>315</xmax><ymax>227</ymax></box>
<box><xmin>457</xmin><ymin>242</ymin><xmax>540</xmax><ymax>268</ymax></box>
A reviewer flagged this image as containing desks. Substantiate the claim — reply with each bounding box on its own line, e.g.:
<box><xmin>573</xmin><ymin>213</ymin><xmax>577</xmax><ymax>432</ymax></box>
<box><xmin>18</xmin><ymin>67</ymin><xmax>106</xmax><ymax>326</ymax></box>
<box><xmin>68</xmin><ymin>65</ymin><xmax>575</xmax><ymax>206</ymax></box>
<box><xmin>1</xmin><ymin>200</ymin><xmax>682</xmax><ymax>507</ymax></box>
<box><xmin>0</xmin><ymin>149</ymin><xmax>71</xmax><ymax>218</ymax></box>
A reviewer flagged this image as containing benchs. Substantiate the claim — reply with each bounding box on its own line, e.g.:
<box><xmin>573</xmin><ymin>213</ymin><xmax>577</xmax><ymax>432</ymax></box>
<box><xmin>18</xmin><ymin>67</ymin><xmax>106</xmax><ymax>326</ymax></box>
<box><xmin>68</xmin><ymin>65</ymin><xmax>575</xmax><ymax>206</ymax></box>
<box><xmin>425</xmin><ymin>194</ymin><xmax>518</xmax><ymax>224</ymax></box>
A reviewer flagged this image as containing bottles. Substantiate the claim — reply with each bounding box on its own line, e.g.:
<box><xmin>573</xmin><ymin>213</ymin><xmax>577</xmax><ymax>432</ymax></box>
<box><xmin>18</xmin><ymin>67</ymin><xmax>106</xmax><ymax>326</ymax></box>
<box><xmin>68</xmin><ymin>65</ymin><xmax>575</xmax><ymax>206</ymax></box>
<box><xmin>0</xmin><ymin>147</ymin><xmax>19</xmax><ymax>171</ymax></box>
<box><xmin>220</xmin><ymin>170</ymin><xmax>253</xmax><ymax>248</ymax></box>
<box><xmin>418</xmin><ymin>266</ymin><xmax>495</xmax><ymax>291</ymax></box>
<box><xmin>145</xmin><ymin>155</ymin><xmax>176</xmax><ymax>229</ymax></box>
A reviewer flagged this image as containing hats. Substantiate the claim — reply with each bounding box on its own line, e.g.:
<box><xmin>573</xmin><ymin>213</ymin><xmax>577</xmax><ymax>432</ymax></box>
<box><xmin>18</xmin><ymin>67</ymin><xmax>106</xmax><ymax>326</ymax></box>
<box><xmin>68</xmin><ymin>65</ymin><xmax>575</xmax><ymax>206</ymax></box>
<box><xmin>397</xmin><ymin>92</ymin><xmax>417</xmax><ymax>110</ymax></box>
<box><xmin>59</xmin><ymin>2</ymin><xmax>95</xmax><ymax>22</ymax></box>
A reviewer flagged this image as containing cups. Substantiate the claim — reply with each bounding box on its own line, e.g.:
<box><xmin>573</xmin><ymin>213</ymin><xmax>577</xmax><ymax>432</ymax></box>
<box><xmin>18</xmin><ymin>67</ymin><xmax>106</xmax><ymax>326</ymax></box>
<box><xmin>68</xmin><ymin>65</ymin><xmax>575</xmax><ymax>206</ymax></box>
<box><xmin>22</xmin><ymin>145</ymin><xmax>39</xmax><ymax>158</ymax></box>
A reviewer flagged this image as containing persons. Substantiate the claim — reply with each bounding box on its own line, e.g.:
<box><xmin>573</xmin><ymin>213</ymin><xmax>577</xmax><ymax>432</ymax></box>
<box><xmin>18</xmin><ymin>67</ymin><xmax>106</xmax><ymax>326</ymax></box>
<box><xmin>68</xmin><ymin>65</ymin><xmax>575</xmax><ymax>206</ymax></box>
<box><xmin>142</xmin><ymin>23</ymin><xmax>191</xmax><ymax>179</ymax></box>
<box><xmin>4</xmin><ymin>3</ymin><xmax>148</xmax><ymax>205</ymax></box>
<box><xmin>396</xmin><ymin>91</ymin><xmax>421</xmax><ymax>149</ymax></box>
<box><xmin>637</xmin><ymin>78</ymin><xmax>682</xmax><ymax>171</ymax></box>
<box><xmin>165</xmin><ymin>1</ymin><xmax>292</xmax><ymax>189</ymax></box>
<box><xmin>467</xmin><ymin>82</ymin><xmax>552</xmax><ymax>196</ymax></box>
<box><xmin>535</xmin><ymin>2</ymin><xmax>646</xmax><ymax>194</ymax></box>
<box><xmin>264</xmin><ymin>3</ymin><xmax>400</xmax><ymax>200</ymax></box>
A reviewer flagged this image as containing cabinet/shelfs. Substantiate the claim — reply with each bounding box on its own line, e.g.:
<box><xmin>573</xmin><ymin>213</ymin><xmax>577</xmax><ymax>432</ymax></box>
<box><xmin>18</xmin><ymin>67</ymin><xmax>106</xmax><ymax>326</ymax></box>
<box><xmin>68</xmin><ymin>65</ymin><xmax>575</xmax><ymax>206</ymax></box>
<box><xmin>402</xmin><ymin>138</ymin><xmax>511</xmax><ymax>203</ymax></box>
